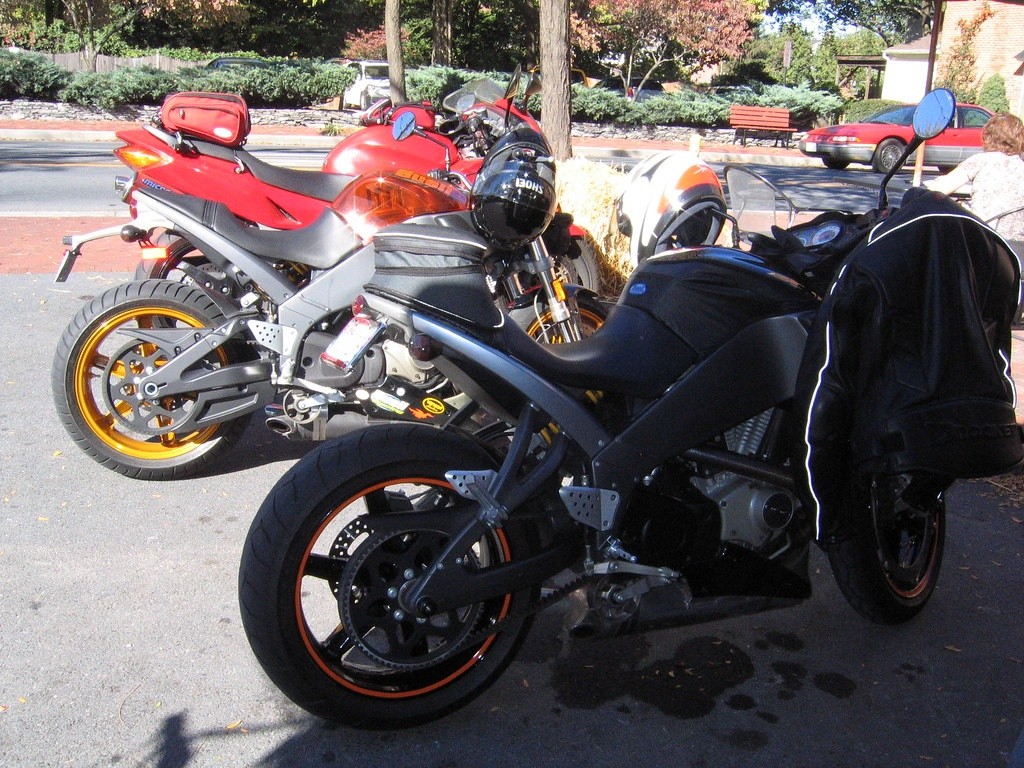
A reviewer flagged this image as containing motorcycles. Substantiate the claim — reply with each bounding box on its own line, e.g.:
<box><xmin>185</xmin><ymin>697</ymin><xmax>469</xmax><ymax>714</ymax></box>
<box><xmin>234</xmin><ymin>89</ymin><xmax>1024</xmax><ymax>729</ymax></box>
<box><xmin>49</xmin><ymin>57</ymin><xmax>636</xmax><ymax>481</ymax></box>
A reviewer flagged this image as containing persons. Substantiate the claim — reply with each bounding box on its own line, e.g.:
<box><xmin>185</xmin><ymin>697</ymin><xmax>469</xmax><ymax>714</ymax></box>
<box><xmin>920</xmin><ymin>112</ymin><xmax>1024</xmax><ymax>331</ymax></box>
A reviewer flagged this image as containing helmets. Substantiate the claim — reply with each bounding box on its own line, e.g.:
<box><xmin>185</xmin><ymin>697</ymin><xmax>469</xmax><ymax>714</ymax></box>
<box><xmin>469</xmin><ymin>128</ymin><xmax>557</xmax><ymax>250</ymax></box>
<box><xmin>616</xmin><ymin>151</ymin><xmax>728</xmax><ymax>270</ymax></box>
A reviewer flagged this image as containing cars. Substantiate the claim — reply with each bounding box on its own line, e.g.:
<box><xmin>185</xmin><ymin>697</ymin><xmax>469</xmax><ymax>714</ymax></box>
<box><xmin>595</xmin><ymin>76</ymin><xmax>666</xmax><ymax>104</ymax></box>
<box><xmin>340</xmin><ymin>61</ymin><xmax>396</xmax><ymax>111</ymax></box>
<box><xmin>523</xmin><ymin>66</ymin><xmax>591</xmax><ymax>108</ymax></box>
<box><xmin>204</xmin><ymin>57</ymin><xmax>269</xmax><ymax>72</ymax></box>
<box><xmin>799</xmin><ymin>102</ymin><xmax>997</xmax><ymax>175</ymax></box>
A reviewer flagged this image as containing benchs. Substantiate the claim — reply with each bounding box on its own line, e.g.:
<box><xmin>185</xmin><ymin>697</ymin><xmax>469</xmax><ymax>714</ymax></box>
<box><xmin>729</xmin><ymin>106</ymin><xmax>798</xmax><ymax>149</ymax></box>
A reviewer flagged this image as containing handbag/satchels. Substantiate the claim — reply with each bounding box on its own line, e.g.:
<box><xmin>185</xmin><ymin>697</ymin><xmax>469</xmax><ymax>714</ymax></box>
<box><xmin>160</xmin><ymin>91</ymin><xmax>252</xmax><ymax>149</ymax></box>
